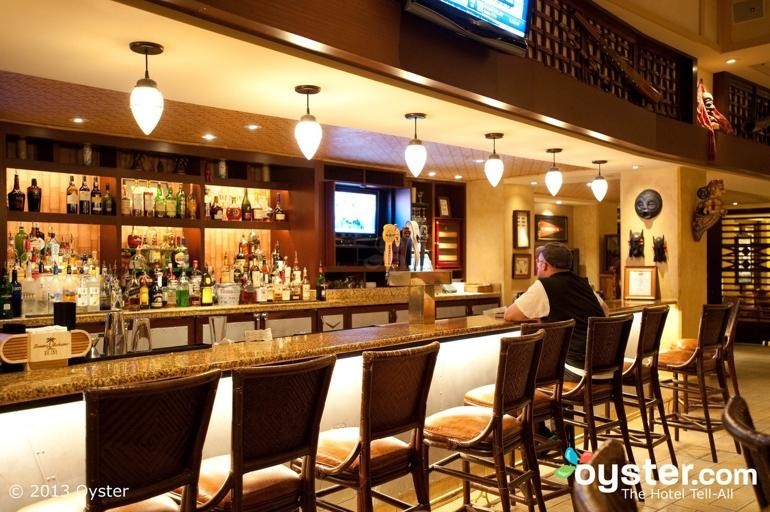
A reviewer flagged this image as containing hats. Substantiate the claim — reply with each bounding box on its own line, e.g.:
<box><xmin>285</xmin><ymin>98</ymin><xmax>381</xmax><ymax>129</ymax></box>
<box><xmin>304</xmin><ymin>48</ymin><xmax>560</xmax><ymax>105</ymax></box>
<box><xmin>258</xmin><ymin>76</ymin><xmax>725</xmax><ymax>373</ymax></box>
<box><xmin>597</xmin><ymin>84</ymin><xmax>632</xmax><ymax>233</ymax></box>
<box><xmin>536</xmin><ymin>241</ymin><xmax>574</xmax><ymax>269</ymax></box>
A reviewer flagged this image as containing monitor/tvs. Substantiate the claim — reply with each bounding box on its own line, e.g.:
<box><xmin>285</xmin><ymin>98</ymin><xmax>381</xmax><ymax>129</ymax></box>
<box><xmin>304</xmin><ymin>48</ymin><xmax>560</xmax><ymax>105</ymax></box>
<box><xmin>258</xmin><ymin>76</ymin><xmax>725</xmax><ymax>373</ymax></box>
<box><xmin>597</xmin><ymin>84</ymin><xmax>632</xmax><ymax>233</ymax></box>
<box><xmin>334</xmin><ymin>184</ymin><xmax>381</xmax><ymax>248</ymax></box>
<box><xmin>406</xmin><ymin>0</ymin><xmax>538</xmax><ymax>59</ymax></box>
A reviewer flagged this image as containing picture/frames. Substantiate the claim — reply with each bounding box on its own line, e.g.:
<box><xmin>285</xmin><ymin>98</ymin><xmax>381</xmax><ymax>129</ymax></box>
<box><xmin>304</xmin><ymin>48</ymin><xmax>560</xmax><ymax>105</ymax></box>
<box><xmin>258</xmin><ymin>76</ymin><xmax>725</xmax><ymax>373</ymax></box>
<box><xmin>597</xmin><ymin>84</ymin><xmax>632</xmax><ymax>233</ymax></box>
<box><xmin>533</xmin><ymin>245</ymin><xmax>580</xmax><ymax>277</ymax></box>
<box><xmin>535</xmin><ymin>215</ymin><xmax>568</xmax><ymax>242</ymax></box>
<box><xmin>437</xmin><ymin>196</ymin><xmax>451</xmax><ymax>217</ymax></box>
<box><xmin>512</xmin><ymin>210</ymin><xmax>531</xmax><ymax>248</ymax></box>
<box><xmin>623</xmin><ymin>266</ymin><xmax>656</xmax><ymax>296</ymax></box>
<box><xmin>512</xmin><ymin>254</ymin><xmax>533</xmax><ymax>272</ymax></box>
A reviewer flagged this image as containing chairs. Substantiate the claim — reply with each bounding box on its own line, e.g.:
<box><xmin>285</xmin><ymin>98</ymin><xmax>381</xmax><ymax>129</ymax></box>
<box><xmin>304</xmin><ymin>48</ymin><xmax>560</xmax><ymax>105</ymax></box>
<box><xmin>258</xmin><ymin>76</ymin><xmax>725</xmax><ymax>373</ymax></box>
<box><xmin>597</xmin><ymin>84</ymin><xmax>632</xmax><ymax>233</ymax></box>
<box><xmin>417</xmin><ymin>328</ymin><xmax>549</xmax><ymax>512</ymax></box>
<box><xmin>580</xmin><ymin>306</ymin><xmax>681</xmax><ymax>483</ymax></box>
<box><xmin>640</xmin><ymin>303</ymin><xmax>743</xmax><ymax>465</ymax></box>
<box><xmin>166</xmin><ymin>355</ymin><xmax>318</xmax><ymax>511</ymax></box>
<box><xmin>538</xmin><ymin>310</ymin><xmax>648</xmax><ymax>505</ymax></box>
<box><xmin>673</xmin><ymin>299</ymin><xmax>745</xmax><ymax>457</ymax></box>
<box><xmin>20</xmin><ymin>373</ymin><xmax>220</xmax><ymax>511</ymax></box>
<box><xmin>721</xmin><ymin>396</ymin><xmax>768</xmax><ymax>511</ymax></box>
<box><xmin>465</xmin><ymin>320</ymin><xmax>577</xmax><ymax>510</ymax></box>
<box><xmin>293</xmin><ymin>340</ymin><xmax>427</xmax><ymax>511</ymax></box>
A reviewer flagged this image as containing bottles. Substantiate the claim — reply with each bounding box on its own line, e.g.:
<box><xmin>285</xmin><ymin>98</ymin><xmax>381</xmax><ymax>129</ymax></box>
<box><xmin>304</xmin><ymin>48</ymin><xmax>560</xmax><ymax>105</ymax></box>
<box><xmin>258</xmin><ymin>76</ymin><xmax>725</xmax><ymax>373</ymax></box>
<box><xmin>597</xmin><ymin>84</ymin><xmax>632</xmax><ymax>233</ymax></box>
<box><xmin>143</xmin><ymin>181</ymin><xmax>155</xmax><ymax>220</ymax></box>
<box><xmin>168</xmin><ymin>281</ymin><xmax>177</xmax><ymax>307</ymax></box>
<box><xmin>273</xmin><ymin>191</ymin><xmax>285</xmax><ymax>220</ymax></box>
<box><xmin>252</xmin><ymin>191</ymin><xmax>264</xmax><ymax>220</ymax></box>
<box><xmin>7</xmin><ymin>222</ymin><xmax>311</xmax><ymax>317</ymax></box>
<box><xmin>64</xmin><ymin>171</ymin><xmax>80</xmax><ymax>213</ymax></box>
<box><xmin>207</xmin><ymin>194</ymin><xmax>223</xmax><ymax>223</ymax></box>
<box><xmin>9</xmin><ymin>269</ymin><xmax>22</xmax><ymax>314</ymax></box>
<box><xmin>154</xmin><ymin>182</ymin><xmax>165</xmax><ymax>220</ymax></box>
<box><xmin>7</xmin><ymin>172</ymin><xmax>25</xmax><ymax>212</ymax></box>
<box><xmin>104</xmin><ymin>183</ymin><xmax>117</xmax><ymax>213</ymax></box>
<box><xmin>132</xmin><ymin>182</ymin><xmax>144</xmax><ymax>222</ymax></box>
<box><xmin>177</xmin><ymin>184</ymin><xmax>186</xmax><ymax>219</ymax></box>
<box><xmin>240</xmin><ymin>185</ymin><xmax>257</xmax><ymax>218</ymax></box>
<box><xmin>92</xmin><ymin>180</ymin><xmax>101</xmax><ymax>214</ymax></box>
<box><xmin>120</xmin><ymin>182</ymin><xmax>134</xmax><ymax>220</ymax></box>
<box><xmin>177</xmin><ymin>272</ymin><xmax>191</xmax><ymax>306</ymax></box>
<box><xmin>164</xmin><ymin>183</ymin><xmax>175</xmax><ymax>215</ymax></box>
<box><xmin>226</xmin><ymin>193</ymin><xmax>241</xmax><ymax>220</ymax></box>
<box><xmin>188</xmin><ymin>182</ymin><xmax>198</xmax><ymax>222</ymax></box>
<box><xmin>139</xmin><ymin>265</ymin><xmax>149</xmax><ymax>307</ymax></box>
<box><xmin>151</xmin><ymin>278</ymin><xmax>166</xmax><ymax>308</ymax></box>
<box><xmin>317</xmin><ymin>256</ymin><xmax>328</xmax><ymax>301</ymax></box>
<box><xmin>27</xmin><ymin>177</ymin><xmax>43</xmax><ymax>212</ymax></box>
<box><xmin>79</xmin><ymin>176</ymin><xmax>93</xmax><ymax>212</ymax></box>
<box><xmin>201</xmin><ymin>264</ymin><xmax>213</xmax><ymax>306</ymax></box>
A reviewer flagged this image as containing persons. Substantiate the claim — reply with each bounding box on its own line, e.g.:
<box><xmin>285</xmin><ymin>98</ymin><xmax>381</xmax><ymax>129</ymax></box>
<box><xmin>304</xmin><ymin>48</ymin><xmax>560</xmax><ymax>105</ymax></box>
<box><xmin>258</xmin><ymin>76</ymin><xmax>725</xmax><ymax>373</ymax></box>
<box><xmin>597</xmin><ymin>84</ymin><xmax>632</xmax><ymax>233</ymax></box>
<box><xmin>504</xmin><ymin>242</ymin><xmax>609</xmax><ymax>458</ymax></box>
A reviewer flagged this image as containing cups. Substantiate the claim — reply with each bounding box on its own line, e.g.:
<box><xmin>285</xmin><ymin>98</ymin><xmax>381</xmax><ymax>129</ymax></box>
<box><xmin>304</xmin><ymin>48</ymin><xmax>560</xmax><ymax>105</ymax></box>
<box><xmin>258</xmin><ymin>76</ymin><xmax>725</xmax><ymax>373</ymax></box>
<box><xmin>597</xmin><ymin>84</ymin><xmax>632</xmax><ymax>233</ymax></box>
<box><xmin>209</xmin><ymin>315</ymin><xmax>227</xmax><ymax>354</ymax></box>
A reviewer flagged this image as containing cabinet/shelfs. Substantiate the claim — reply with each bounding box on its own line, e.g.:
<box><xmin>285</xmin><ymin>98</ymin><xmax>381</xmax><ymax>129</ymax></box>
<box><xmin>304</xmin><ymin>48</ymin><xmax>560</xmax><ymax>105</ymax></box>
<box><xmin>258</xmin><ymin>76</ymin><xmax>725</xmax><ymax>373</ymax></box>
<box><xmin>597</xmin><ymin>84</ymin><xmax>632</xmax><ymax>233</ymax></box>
<box><xmin>76</xmin><ymin>317</ymin><xmax>198</xmax><ymax>362</ymax></box>
<box><xmin>317</xmin><ymin>159</ymin><xmax>499</xmax><ymax>307</ymax></box>
<box><xmin>347</xmin><ymin>303</ymin><xmax>409</xmax><ymax>329</ymax></box>
<box><xmin>434</xmin><ymin>299</ymin><xmax>503</xmax><ymax>319</ymax></box>
<box><xmin>0</xmin><ymin>121</ymin><xmax>324</xmax><ymax>325</ymax></box>
<box><xmin>316</xmin><ymin>309</ymin><xmax>348</xmax><ymax>333</ymax></box>
<box><xmin>197</xmin><ymin>308</ymin><xmax>314</xmax><ymax>343</ymax></box>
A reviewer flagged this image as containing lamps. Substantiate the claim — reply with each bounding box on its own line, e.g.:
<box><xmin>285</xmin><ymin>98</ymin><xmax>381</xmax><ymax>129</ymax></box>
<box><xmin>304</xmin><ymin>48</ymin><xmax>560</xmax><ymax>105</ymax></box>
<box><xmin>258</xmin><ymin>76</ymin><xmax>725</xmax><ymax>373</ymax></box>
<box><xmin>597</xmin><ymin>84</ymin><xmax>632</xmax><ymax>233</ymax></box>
<box><xmin>127</xmin><ymin>39</ymin><xmax>165</xmax><ymax>134</ymax></box>
<box><xmin>545</xmin><ymin>147</ymin><xmax>565</xmax><ymax>197</ymax></box>
<box><xmin>483</xmin><ymin>132</ymin><xmax>505</xmax><ymax>188</ymax></box>
<box><xmin>405</xmin><ymin>112</ymin><xmax>427</xmax><ymax>178</ymax></box>
<box><xmin>591</xmin><ymin>160</ymin><xmax>608</xmax><ymax>203</ymax></box>
<box><xmin>293</xmin><ymin>84</ymin><xmax>324</xmax><ymax>162</ymax></box>
